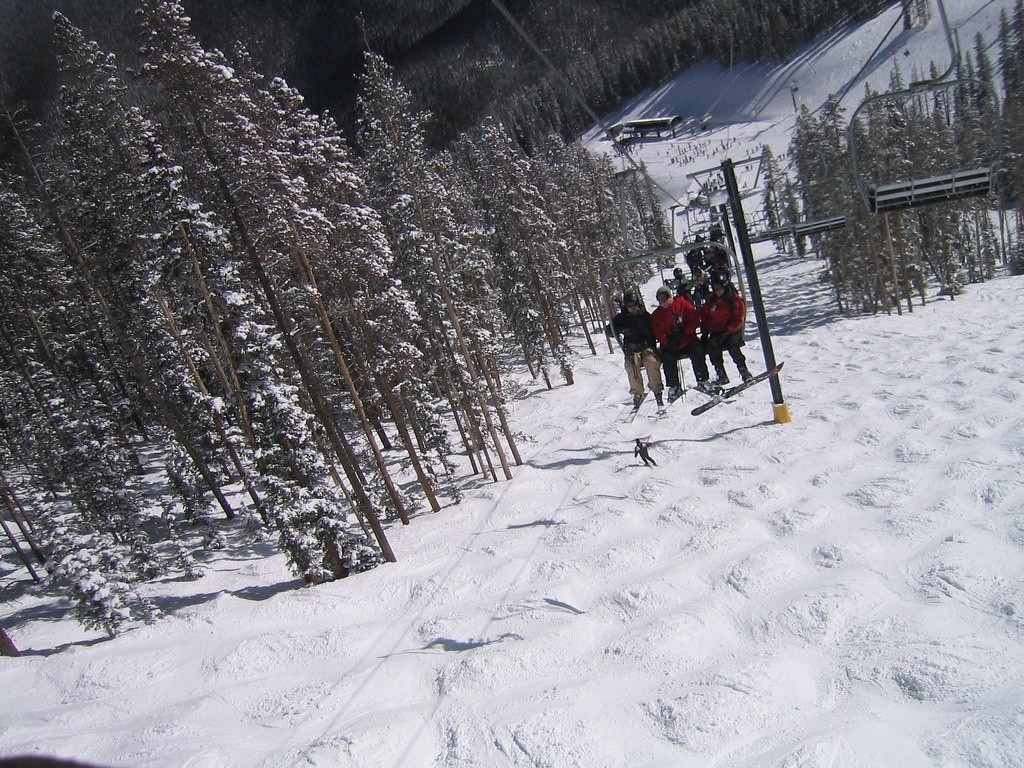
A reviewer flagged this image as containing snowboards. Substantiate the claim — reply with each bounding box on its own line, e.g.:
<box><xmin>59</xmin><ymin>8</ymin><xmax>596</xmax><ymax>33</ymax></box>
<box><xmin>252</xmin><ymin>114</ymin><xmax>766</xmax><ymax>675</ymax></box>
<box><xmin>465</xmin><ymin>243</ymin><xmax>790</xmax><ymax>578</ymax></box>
<box><xmin>692</xmin><ymin>360</ymin><xmax>785</xmax><ymax>416</ymax></box>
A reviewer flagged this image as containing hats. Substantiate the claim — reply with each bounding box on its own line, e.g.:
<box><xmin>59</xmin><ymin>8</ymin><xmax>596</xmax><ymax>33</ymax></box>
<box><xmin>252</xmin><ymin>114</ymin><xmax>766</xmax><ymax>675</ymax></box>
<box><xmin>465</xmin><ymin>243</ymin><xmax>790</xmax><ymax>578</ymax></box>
<box><xmin>673</xmin><ymin>268</ymin><xmax>682</xmax><ymax>273</ymax></box>
<box><xmin>692</xmin><ymin>265</ymin><xmax>701</xmax><ymax>275</ymax></box>
<box><xmin>711</xmin><ymin>272</ymin><xmax>729</xmax><ymax>288</ymax></box>
<box><xmin>624</xmin><ymin>292</ymin><xmax>639</xmax><ymax>306</ymax></box>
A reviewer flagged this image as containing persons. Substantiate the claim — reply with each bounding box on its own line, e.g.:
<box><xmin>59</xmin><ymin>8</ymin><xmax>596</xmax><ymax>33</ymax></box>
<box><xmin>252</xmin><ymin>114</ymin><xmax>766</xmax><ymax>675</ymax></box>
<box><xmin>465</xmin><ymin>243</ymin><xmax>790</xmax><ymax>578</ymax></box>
<box><xmin>604</xmin><ymin>291</ymin><xmax>664</xmax><ymax>408</ymax></box>
<box><xmin>669</xmin><ymin>265</ymin><xmax>731</xmax><ymax>299</ymax></box>
<box><xmin>686</xmin><ymin>223</ymin><xmax>726</xmax><ymax>269</ymax></box>
<box><xmin>700</xmin><ymin>274</ymin><xmax>753</xmax><ymax>387</ymax></box>
<box><xmin>744</xmin><ymin>142</ymin><xmax>788</xmax><ymax>188</ymax></box>
<box><xmin>707</xmin><ymin>136</ymin><xmax>741</xmax><ymax>160</ymax></box>
<box><xmin>633</xmin><ymin>438</ymin><xmax>656</xmax><ymax>466</ymax></box>
<box><xmin>619</xmin><ymin>134</ymin><xmax>643</xmax><ymax>157</ymax></box>
<box><xmin>655</xmin><ymin>137</ymin><xmax>706</xmax><ymax>166</ymax></box>
<box><xmin>650</xmin><ymin>286</ymin><xmax>715</xmax><ymax>399</ymax></box>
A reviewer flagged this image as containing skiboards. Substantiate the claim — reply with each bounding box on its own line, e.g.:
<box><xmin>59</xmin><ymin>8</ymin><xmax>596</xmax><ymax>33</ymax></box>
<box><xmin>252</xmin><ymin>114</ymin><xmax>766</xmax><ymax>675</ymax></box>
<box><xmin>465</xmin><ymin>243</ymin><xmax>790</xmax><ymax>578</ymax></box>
<box><xmin>654</xmin><ymin>383</ymin><xmax>737</xmax><ymax>421</ymax></box>
<box><xmin>643</xmin><ymin>463</ymin><xmax>659</xmax><ymax>467</ymax></box>
<box><xmin>624</xmin><ymin>379</ymin><xmax>652</xmax><ymax>425</ymax></box>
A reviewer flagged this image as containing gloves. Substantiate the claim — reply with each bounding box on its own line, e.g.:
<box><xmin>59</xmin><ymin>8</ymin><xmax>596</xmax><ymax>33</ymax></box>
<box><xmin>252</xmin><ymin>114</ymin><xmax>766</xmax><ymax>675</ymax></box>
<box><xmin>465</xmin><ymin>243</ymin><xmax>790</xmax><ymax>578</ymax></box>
<box><xmin>646</xmin><ymin>348</ymin><xmax>654</xmax><ymax>354</ymax></box>
<box><xmin>700</xmin><ymin>333</ymin><xmax>709</xmax><ymax>345</ymax></box>
<box><xmin>667</xmin><ymin>327</ymin><xmax>684</xmax><ymax>343</ymax></box>
<box><xmin>619</xmin><ymin>333</ymin><xmax>625</xmax><ymax>342</ymax></box>
<box><xmin>717</xmin><ymin>332</ymin><xmax>727</xmax><ymax>345</ymax></box>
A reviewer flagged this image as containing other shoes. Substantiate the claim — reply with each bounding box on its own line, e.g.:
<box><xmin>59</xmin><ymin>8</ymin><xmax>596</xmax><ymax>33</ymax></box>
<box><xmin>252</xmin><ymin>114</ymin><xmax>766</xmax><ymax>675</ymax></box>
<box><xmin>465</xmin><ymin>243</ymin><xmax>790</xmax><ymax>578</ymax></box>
<box><xmin>654</xmin><ymin>392</ymin><xmax>663</xmax><ymax>406</ymax></box>
<box><xmin>697</xmin><ymin>380</ymin><xmax>714</xmax><ymax>391</ymax></box>
<box><xmin>711</xmin><ymin>378</ymin><xmax>730</xmax><ymax>386</ymax></box>
<box><xmin>632</xmin><ymin>394</ymin><xmax>644</xmax><ymax>405</ymax></box>
<box><xmin>742</xmin><ymin>372</ymin><xmax>753</xmax><ymax>384</ymax></box>
<box><xmin>667</xmin><ymin>383</ymin><xmax>683</xmax><ymax>402</ymax></box>
